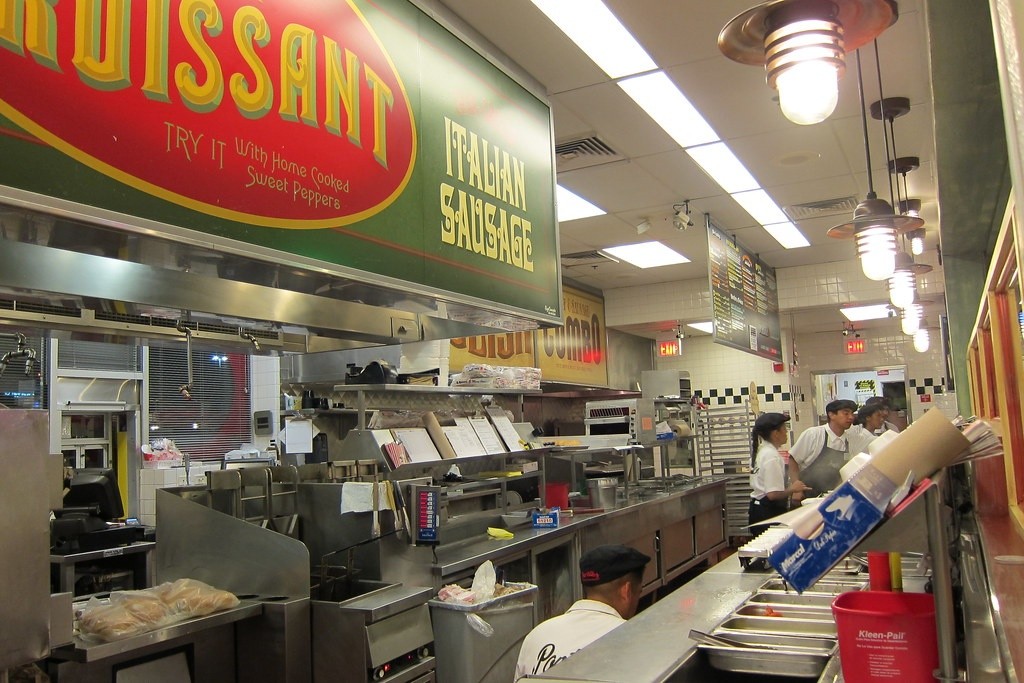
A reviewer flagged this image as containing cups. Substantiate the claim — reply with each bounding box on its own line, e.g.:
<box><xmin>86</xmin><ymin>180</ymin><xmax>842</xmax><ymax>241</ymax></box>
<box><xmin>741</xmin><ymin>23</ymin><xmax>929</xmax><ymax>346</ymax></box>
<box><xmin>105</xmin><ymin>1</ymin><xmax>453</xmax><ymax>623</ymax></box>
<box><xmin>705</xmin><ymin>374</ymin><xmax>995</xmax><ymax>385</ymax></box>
<box><xmin>286</xmin><ymin>396</ymin><xmax>303</xmax><ymax>411</ymax></box>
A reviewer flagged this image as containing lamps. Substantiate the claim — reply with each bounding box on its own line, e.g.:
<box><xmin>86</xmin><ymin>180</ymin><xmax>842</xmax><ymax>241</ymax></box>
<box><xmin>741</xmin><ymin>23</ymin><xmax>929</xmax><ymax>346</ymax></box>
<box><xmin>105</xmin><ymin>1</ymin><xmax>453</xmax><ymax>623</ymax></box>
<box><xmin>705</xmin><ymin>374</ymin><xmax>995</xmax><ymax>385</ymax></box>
<box><xmin>897</xmin><ymin>199</ymin><xmax>923</xmax><ymax>227</ymax></box>
<box><xmin>672</xmin><ymin>201</ymin><xmax>692</xmax><ymax>232</ymax></box>
<box><xmin>870</xmin><ymin>97</ymin><xmax>937</xmax><ymax>336</ymax></box>
<box><xmin>827</xmin><ymin>49</ymin><xmax>925</xmax><ymax>281</ymax></box>
<box><xmin>872</xmin><ymin>37</ymin><xmax>934</xmax><ymax>309</ymax></box>
<box><xmin>887</xmin><ymin>157</ymin><xmax>940</xmax><ymax>352</ymax></box>
<box><xmin>717</xmin><ymin>0</ymin><xmax>899</xmax><ymax>126</ymax></box>
<box><xmin>905</xmin><ymin>228</ymin><xmax>927</xmax><ymax>254</ymax></box>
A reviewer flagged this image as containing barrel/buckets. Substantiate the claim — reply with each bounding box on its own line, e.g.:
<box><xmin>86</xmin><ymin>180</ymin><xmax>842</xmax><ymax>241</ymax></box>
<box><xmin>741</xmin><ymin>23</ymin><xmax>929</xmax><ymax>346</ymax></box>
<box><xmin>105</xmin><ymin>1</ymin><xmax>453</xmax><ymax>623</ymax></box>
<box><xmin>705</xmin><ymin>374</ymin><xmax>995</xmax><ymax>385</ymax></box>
<box><xmin>538</xmin><ymin>481</ymin><xmax>569</xmax><ymax>510</ymax></box>
<box><xmin>587</xmin><ymin>477</ymin><xmax>619</xmax><ymax>510</ymax></box>
<box><xmin>830</xmin><ymin>590</ymin><xmax>942</xmax><ymax>683</ymax></box>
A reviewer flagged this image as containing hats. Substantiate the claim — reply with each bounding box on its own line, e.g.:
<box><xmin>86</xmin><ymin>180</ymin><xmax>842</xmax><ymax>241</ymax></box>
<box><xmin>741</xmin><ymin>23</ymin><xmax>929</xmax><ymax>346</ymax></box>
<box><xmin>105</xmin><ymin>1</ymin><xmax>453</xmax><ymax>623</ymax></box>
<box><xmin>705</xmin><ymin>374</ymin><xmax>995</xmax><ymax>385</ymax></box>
<box><xmin>826</xmin><ymin>400</ymin><xmax>858</xmax><ymax>413</ymax></box>
<box><xmin>580</xmin><ymin>544</ymin><xmax>650</xmax><ymax>586</ymax></box>
<box><xmin>755</xmin><ymin>413</ymin><xmax>790</xmax><ymax>430</ymax></box>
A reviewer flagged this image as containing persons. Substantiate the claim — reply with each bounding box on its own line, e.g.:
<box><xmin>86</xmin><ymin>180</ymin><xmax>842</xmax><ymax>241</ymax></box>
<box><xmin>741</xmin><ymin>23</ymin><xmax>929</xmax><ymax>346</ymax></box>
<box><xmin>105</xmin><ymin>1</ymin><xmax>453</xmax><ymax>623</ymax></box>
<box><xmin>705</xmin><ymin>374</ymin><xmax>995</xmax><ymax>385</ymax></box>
<box><xmin>847</xmin><ymin>396</ymin><xmax>900</xmax><ymax>457</ymax></box>
<box><xmin>514</xmin><ymin>544</ymin><xmax>651</xmax><ymax>683</ymax></box>
<box><xmin>787</xmin><ymin>400</ymin><xmax>858</xmax><ymax>511</ymax></box>
<box><xmin>748</xmin><ymin>412</ymin><xmax>812</xmax><ymax>536</ymax></box>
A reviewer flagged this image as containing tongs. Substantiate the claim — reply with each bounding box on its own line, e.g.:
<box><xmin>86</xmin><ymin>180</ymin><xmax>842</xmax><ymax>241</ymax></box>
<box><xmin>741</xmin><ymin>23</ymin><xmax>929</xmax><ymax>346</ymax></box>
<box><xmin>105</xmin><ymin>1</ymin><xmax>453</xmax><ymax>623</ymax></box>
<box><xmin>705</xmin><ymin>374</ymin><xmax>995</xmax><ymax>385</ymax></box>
<box><xmin>687</xmin><ymin>628</ymin><xmax>778</xmax><ymax>651</ymax></box>
<box><xmin>370</xmin><ymin>482</ymin><xmax>381</xmax><ymax>539</ymax></box>
<box><xmin>382</xmin><ymin>480</ymin><xmax>412</xmax><ymax>540</ymax></box>
